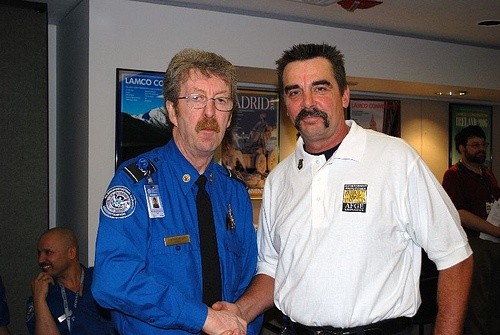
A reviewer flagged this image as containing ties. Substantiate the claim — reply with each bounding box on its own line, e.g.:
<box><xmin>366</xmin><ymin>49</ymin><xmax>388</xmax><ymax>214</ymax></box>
<box><xmin>195</xmin><ymin>175</ymin><xmax>223</xmax><ymax>335</ymax></box>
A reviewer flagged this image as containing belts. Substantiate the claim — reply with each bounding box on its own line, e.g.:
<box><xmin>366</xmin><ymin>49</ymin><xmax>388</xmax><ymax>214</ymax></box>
<box><xmin>273</xmin><ymin>302</ymin><xmax>409</xmax><ymax>335</ymax></box>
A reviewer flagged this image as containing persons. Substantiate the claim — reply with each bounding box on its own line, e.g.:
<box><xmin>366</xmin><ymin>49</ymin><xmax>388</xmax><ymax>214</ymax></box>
<box><xmin>211</xmin><ymin>42</ymin><xmax>475</xmax><ymax>335</ymax></box>
<box><xmin>443</xmin><ymin>125</ymin><xmax>500</xmax><ymax>335</ymax></box>
<box><xmin>91</xmin><ymin>49</ymin><xmax>264</xmax><ymax>335</ymax></box>
<box><xmin>24</xmin><ymin>226</ymin><xmax>119</xmax><ymax>335</ymax></box>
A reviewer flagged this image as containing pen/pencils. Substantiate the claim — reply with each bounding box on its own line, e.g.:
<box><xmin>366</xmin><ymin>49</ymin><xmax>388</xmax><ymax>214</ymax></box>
<box><xmin>226</xmin><ymin>204</ymin><xmax>236</xmax><ymax>231</ymax></box>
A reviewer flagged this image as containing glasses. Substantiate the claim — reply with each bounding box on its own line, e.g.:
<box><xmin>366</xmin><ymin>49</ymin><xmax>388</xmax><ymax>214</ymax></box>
<box><xmin>466</xmin><ymin>142</ymin><xmax>489</xmax><ymax>149</ymax></box>
<box><xmin>177</xmin><ymin>93</ymin><xmax>236</xmax><ymax>112</ymax></box>
